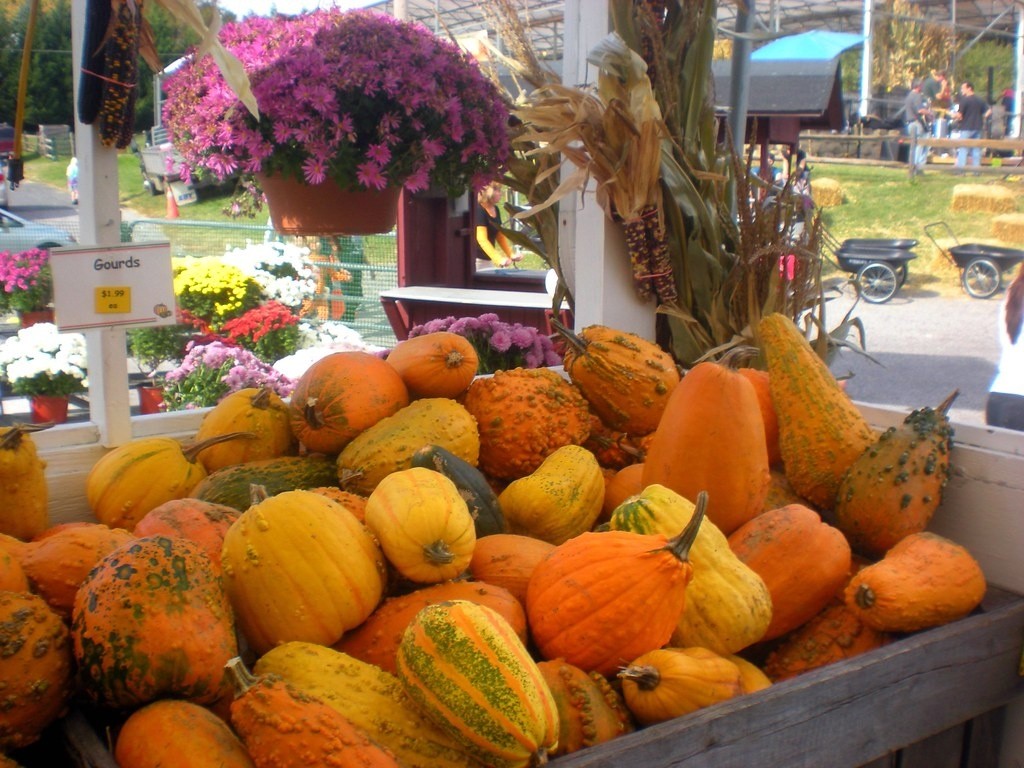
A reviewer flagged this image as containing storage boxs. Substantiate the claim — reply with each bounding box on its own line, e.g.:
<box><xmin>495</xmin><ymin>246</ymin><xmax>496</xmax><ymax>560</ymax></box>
<box><xmin>65</xmin><ymin>583</ymin><xmax>1024</xmax><ymax>768</ymax></box>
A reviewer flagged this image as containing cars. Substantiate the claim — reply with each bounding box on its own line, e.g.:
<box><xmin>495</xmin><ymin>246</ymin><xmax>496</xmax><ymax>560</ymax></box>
<box><xmin>0</xmin><ymin>167</ymin><xmax>9</xmax><ymax>216</ymax></box>
<box><xmin>729</xmin><ymin>164</ymin><xmax>813</xmax><ymax>230</ymax></box>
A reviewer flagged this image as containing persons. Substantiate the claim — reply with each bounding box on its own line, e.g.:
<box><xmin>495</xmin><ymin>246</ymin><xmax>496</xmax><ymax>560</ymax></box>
<box><xmin>66</xmin><ymin>158</ymin><xmax>79</xmax><ymax>204</ymax></box>
<box><xmin>906</xmin><ymin>83</ymin><xmax>934</xmax><ymax>176</ymax></box>
<box><xmin>984</xmin><ymin>263</ymin><xmax>1024</xmax><ymax>431</ymax></box>
<box><xmin>936</xmin><ymin>72</ymin><xmax>952</xmax><ymax>108</ymax></box>
<box><xmin>952</xmin><ymin>81</ymin><xmax>992</xmax><ymax>167</ymax></box>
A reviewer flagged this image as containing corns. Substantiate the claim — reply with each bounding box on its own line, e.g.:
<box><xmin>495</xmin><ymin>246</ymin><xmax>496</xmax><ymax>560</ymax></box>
<box><xmin>622</xmin><ymin>205</ymin><xmax>678</xmax><ymax>306</ymax></box>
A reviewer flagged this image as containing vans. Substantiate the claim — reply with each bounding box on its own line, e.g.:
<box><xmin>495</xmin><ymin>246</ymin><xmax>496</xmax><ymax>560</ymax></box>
<box><xmin>0</xmin><ymin>123</ymin><xmax>15</xmax><ymax>168</ymax></box>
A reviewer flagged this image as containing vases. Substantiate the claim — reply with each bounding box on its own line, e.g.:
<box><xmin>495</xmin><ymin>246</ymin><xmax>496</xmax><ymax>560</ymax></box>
<box><xmin>22</xmin><ymin>308</ymin><xmax>54</xmax><ymax>328</ymax></box>
<box><xmin>30</xmin><ymin>393</ymin><xmax>68</xmax><ymax>425</ymax></box>
<box><xmin>138</xmin><ymin>381</ymin><xmax>165</xmax><ymax>414</ymax></box>
<box><xmin>254</xmin><ymin>167</ymin><xmax>401</xmax><ymax>237</ymax></box>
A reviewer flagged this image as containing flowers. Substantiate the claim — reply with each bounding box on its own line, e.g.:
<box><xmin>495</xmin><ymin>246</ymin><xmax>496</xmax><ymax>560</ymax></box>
<box><xmin>0</xmin><ymin>245</ymin><xmax>560</xmax><ymax>396</ymax></box>
<box><xmin>163</xmin><ymin>6</ymin><xmax>509</xmax><ymax>197</ymax></box>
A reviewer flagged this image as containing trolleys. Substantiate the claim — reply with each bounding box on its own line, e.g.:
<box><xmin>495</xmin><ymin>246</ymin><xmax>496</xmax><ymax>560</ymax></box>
<box><xmin>818</xmin><ymin>224</ymin><xmax>919</xmax><ymax>305</ymax></box>
<box><xmin>924</xmin><ymin>221</ymin><xmax>1024</xmax><ymax>299</ymax></box>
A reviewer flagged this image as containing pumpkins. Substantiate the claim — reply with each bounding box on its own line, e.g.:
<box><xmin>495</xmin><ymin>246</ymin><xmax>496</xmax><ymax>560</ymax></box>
<box><xmin>0</xmin><ymin>317</ymin><xmax>988</xmax><ymax>767</ymax></box>
